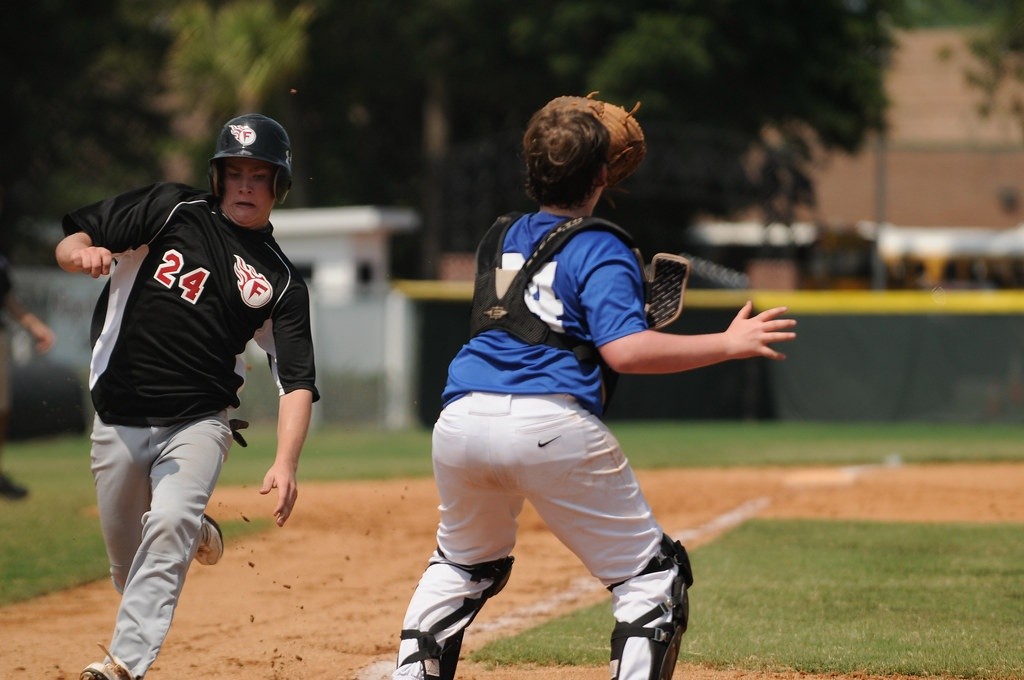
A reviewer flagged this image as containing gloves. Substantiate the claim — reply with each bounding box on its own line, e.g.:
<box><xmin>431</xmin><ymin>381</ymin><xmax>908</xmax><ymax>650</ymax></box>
<box><xmin>229</xmin><ymin>419</ymin><xmax>249</xmax><ymax>448</ymax></box>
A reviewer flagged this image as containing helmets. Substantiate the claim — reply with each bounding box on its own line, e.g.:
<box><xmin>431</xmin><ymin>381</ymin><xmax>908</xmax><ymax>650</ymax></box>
<box><xmin>206</xmin><ymin>113</ymin><xmax>292</xmax><ymax>205</ymax></box>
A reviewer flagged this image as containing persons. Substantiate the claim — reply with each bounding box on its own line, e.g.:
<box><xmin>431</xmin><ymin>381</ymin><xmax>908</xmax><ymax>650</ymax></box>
<box><xmin>395</xmin><ymin>93</ymin><xmax>799</xmax><ymax>679</ymax></box>
<box><xmin>1</xmin><ymin>256</ymin><xmax>59</xmax><ymax>499</ymax></box>
<box><xmin>50</xmin><ymin>112</ymin><xmax>321</xmax><ymax>679</ymax></box>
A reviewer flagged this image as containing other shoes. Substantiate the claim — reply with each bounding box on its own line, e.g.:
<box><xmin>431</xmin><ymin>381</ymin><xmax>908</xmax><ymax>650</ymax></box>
<box><xmin>0</xmin><ymin>473</ymin><xmax>25</xmax><ymax>498</ymax></box>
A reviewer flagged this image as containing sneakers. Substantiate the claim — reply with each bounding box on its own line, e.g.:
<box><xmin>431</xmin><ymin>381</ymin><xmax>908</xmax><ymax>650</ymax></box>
<box><xmin>80</xmin><ymin>642</ymin><xmax>132</xmax><ymax>680</ymax></box>
<box><xmin>192</xmin><ymin>514</ymin><xmax>223</xmax><ymax>566</ymax></box>
